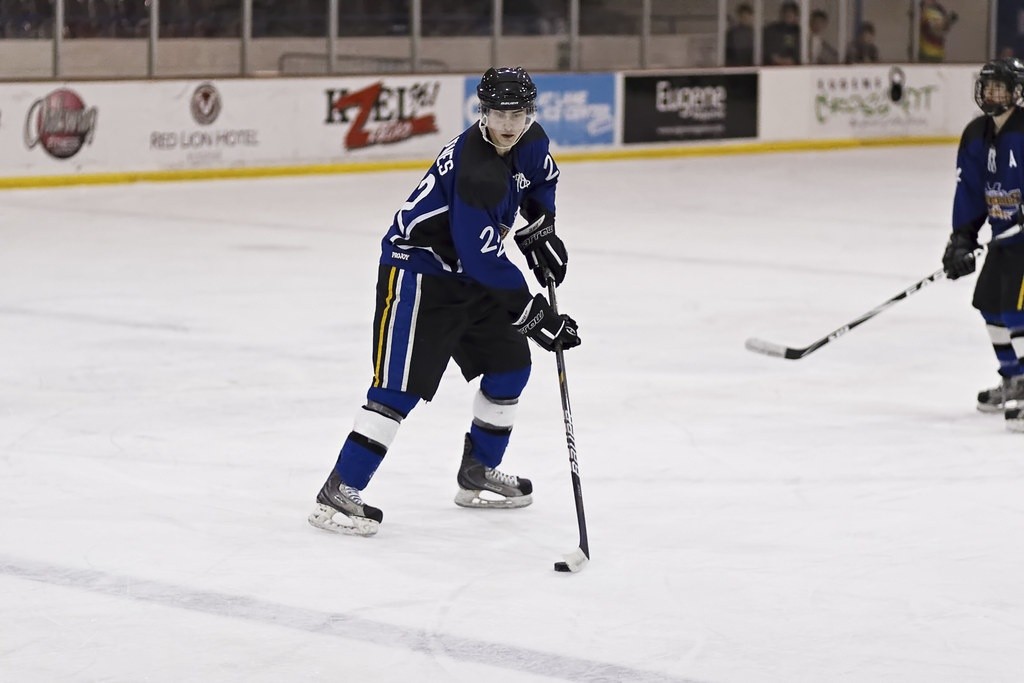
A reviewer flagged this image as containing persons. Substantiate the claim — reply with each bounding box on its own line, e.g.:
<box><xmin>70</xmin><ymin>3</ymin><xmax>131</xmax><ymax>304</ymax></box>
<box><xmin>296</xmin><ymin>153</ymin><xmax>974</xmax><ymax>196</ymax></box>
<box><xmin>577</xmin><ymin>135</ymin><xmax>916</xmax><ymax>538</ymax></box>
<box><xmin>308</xmin><ymin>67</ymin><xmax>580</xmax><ymax>536</ymax></box>
<box><xmin>723</xmin><ymin>0</ymin><xmax>960</xmax><ymax>66</ymax></box>
<box><xmin>943</xmin><ymin>57</ymin><xmax>1024</xmax><ymax>433</ymax></box>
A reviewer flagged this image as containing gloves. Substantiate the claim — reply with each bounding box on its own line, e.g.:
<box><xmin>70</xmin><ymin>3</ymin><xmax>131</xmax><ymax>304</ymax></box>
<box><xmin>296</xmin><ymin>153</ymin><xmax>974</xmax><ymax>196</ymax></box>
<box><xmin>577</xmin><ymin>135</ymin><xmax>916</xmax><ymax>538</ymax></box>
<box><xmin>943</xmin><ymin>227</ymin><xmax>984</xmax><ymax>280</ymax></box>
<box><xmin>514</xmin><ymin>212</ymin><xmax>568</xmax><ymax>288</ymax></box>
<box><xmin>510</xmin><ymin>293</ymin><xmax>581</xmax><ymax>352</ymax></box>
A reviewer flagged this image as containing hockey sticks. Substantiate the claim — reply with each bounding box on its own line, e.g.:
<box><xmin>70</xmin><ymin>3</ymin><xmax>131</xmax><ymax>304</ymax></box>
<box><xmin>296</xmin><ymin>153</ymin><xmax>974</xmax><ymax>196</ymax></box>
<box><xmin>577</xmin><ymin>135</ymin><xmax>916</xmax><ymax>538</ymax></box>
<box><xmin>544</xmin><ymin>277</ymin><xmax>590</xmax><ymax>571</ymax></box>
<box><xmin>745</xmin><ymin>225</ymin><xmax>1020</xmax><ymax>360</ymax></box>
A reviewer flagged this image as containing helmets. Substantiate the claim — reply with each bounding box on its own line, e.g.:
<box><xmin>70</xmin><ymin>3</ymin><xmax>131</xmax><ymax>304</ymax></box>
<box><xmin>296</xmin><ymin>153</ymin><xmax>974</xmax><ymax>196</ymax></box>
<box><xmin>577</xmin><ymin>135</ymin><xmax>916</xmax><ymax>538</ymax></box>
<box><xmin>981</xmin><ymin>57</ymin><xmax>1024</xmax><ymax>87</ymax></box>
<box><xmin>477</xmin><ymin>66</ymin><xmax>537</xmax><ymax>111</ymax></box>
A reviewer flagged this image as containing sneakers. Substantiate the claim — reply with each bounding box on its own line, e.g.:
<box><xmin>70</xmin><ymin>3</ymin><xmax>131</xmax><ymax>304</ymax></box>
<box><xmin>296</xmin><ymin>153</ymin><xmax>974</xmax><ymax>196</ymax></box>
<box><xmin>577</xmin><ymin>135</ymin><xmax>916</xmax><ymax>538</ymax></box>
<box><xmin>1004</xmin><ymin>409</ymin><xmax>1024</xmax><ymax>432</ymax></box>
<box><xmin>977</xmin><ymin>364</ymin><xmax>1024</xmax><ymax>412</ymax></box>
<box><xmin>454</xmin><ymin>432</ymin><xmax>532</xmax><ymax>509</ymax></box>
<box><xmin>309</xmin><ymin>467</ymin><xmax>383</xmax><ymax>536</ymax></box>
